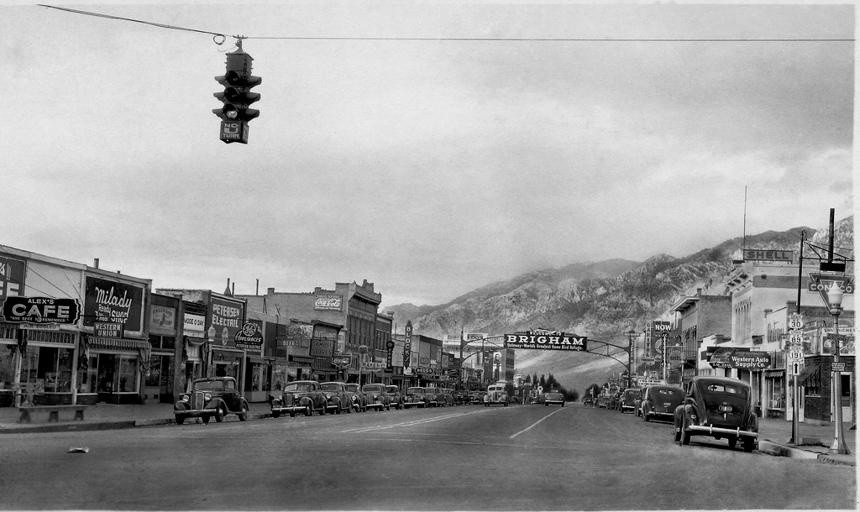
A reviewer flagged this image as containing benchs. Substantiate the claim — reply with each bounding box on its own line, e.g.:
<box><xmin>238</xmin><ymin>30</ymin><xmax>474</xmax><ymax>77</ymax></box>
<box><xmin>18</xmin><ymin>401</ymin><xmax>88</xmax><ymax>422</ymax></box>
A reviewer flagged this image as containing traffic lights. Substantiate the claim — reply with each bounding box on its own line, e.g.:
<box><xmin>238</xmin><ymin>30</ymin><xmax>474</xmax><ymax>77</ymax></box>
<box><xmin>212</xmin><ymin>51</ymin><xmax>262</xmax><ymax>144</ymax></box>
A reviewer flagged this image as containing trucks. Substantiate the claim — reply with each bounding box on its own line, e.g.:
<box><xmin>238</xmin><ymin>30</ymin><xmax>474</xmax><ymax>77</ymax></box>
<box><xmin>483</xmin><ymin>379</ymin><xmax>523</xmax><ymax>407</ymax></box>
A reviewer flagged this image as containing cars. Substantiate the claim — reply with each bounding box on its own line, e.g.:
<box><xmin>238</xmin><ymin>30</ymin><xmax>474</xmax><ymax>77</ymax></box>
<box><xmin>544</xmin><ymin>389</ymin><xmax>565</xmax><ymax>407</ymax></box>
<box><xmin>173</xmin><ymin>376</ymin><xmax>249</xmax><ymax>424</ymax></box>
<box><xmin>44</xmin><ymin>369</ymin><xmax>81</xmax><ymax>392</ymax></box>
<box><xmin>583</xmin><ymin>376</ymin><xmax>758</xmax><ymax>453</ymax></box>
<box><xmin>271</xmin><ymin>380</ymin><xmax>487</xmax><ymax>418</ymax></box>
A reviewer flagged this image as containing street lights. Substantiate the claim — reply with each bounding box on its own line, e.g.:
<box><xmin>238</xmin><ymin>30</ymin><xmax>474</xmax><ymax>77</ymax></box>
<box><xmin>828</xmin><ymin>281</ymin><xmax>849</xmax><ymax>453</ymax></box>
<box><xmin>207</xmin><ymin>324</ymin><xmax>216</xmax><ymax>378</ymax></box>
<box><xmin>680</xmin><ymin>352</ymin><xmax>687</xmax><ymax>376</ymax></box>
